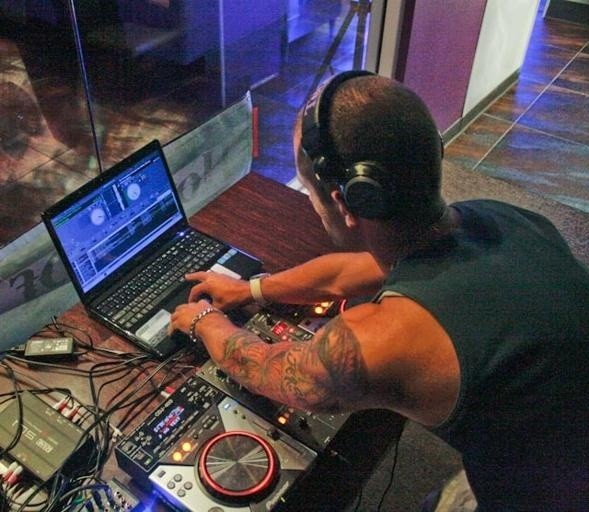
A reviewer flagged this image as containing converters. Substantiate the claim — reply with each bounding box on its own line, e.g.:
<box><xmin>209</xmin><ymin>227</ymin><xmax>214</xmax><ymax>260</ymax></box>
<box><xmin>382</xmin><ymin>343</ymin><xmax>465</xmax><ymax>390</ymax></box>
<box><xmin>24</xmin><ymin>337</ymin><xmax>77</xmax><ymax>363</ymax></box>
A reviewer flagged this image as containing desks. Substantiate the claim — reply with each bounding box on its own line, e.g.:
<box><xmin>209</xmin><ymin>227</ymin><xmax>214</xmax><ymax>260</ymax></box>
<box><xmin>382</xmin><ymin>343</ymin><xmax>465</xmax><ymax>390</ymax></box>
<box><xmin>0</xmin><ymin>167</ymin><xmax>408</xmax><ymax>511</ymax></box>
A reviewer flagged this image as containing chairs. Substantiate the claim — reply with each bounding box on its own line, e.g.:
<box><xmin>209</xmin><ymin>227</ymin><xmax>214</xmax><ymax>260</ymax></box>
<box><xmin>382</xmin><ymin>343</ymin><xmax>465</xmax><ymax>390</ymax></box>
<box><xmin>53</xmin><ymin>0</ymin><xmax>178</xmax><ymax>102</ymax></box>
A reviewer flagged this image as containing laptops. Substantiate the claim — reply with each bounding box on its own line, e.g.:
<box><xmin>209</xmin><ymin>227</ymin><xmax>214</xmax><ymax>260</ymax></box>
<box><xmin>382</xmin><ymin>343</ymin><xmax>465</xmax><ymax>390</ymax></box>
<box><xmin>40</xmin><ymin>139</ymin><xmax>263</xmax><ymax>363</ymax></box>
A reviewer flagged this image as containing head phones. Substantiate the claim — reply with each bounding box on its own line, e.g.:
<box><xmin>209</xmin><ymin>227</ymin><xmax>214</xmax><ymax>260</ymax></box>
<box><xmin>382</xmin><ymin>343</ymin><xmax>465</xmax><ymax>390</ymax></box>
<box><xmin>300</xmin><ymin>69</ymin><xmax>444</xmax><ymax>220</ymax></box>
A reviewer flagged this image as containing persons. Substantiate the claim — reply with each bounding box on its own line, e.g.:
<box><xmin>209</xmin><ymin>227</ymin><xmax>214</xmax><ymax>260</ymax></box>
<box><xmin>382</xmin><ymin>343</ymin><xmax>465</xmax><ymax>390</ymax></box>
<box><xmin>165</xmin><ymin>71</ymin><xmax>587</xmax><ymax>510</ymax></box>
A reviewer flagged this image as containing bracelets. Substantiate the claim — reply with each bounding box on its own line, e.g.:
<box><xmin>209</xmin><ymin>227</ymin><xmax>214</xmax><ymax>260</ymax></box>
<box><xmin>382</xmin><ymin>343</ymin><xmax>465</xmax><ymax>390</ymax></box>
<box><xmin>249</xmin><ymin>271</ymin><xmax>272</xmax><ymax>307</ymax></box>
<box><xmin>188</xmin><ymin>306</ymin><xmax>224</xmax><ymax>343</ymax></box>
